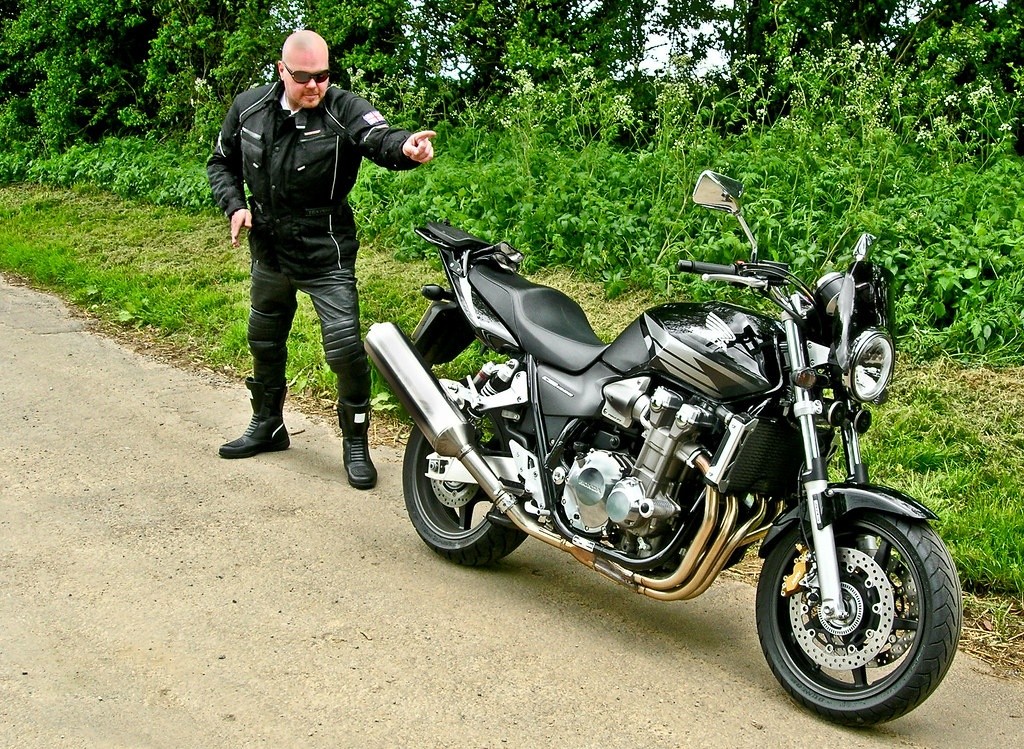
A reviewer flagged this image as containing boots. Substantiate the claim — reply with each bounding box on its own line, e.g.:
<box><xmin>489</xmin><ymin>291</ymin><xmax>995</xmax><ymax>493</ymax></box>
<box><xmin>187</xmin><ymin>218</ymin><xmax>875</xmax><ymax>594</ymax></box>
<box><xmin>219</xmin><ymin>376</ymin><xmax>290</xmax><ymax>459</ymax></box>
<box><xmin>337</xmin><ymin>399</ymin><xmax>378</xmax><ymax>489</ymax></box>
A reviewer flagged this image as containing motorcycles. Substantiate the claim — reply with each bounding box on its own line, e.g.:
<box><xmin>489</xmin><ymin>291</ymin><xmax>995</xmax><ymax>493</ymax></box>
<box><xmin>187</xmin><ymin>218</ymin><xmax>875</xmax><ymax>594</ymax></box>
<box><xmin>363</xmin><ymin>170</ymin><xmax>964</xmax><ymax>730</ymax></box>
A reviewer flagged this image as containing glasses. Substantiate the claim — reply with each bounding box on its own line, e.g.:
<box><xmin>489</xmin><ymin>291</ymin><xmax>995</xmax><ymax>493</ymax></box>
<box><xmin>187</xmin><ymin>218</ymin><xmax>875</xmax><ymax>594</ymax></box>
<box><xmin>283</xmin><ymin>62</ymin><xmax>332</xmax><ymax>83</ymax></box>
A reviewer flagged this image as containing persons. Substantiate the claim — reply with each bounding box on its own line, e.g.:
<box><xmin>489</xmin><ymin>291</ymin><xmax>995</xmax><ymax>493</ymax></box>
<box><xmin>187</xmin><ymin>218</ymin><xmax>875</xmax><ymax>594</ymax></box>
<box><xmin>207</xmin><ymin>30</ymin><xmax>437</xmax><ymax>488</ymax></box>
<box><xmin>721</xmin><ymin>193</ymin><xmax>732</xmax><ymax>202</ymax></box>
